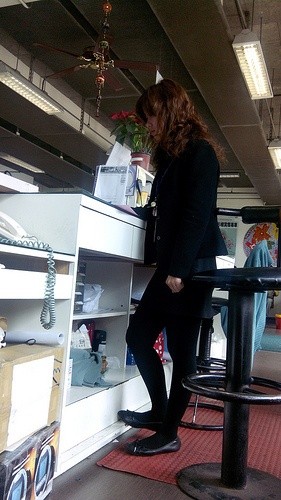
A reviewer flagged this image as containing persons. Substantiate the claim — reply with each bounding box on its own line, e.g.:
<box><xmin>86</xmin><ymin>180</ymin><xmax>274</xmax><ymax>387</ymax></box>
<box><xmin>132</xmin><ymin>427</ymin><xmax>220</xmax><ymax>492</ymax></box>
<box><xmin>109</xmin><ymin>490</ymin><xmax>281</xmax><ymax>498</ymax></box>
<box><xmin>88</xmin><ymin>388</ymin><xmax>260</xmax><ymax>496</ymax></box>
<box><xmin>116</xmin><ymin>80</ymin><xmax>228</xmax><ymax>456</ymax></box>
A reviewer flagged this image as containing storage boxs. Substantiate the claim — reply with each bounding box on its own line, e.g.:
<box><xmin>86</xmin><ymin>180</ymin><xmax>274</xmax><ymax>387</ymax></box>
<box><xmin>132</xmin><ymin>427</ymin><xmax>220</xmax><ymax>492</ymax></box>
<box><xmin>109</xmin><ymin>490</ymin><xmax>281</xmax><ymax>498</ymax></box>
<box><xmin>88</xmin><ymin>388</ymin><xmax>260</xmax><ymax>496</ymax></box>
<box><xmin>0</xmin><ymin>341</ymin><xmax>64</xmax><ymax>453</ymax></box>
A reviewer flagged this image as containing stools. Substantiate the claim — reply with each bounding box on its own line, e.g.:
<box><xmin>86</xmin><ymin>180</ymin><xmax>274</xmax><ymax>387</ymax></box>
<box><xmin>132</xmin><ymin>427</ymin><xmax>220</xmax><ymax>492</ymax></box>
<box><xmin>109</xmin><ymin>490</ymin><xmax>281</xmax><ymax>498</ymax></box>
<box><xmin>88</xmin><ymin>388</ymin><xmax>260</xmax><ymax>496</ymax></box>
<box><xmin>174</xmin><ymin>206</ymin><xmax>281</xmax><ymax>500</ymax></box>
<box><xmin>178</xmin><ymin>298</ymin><xmax>228</xmax><ymax>432</ymax></box>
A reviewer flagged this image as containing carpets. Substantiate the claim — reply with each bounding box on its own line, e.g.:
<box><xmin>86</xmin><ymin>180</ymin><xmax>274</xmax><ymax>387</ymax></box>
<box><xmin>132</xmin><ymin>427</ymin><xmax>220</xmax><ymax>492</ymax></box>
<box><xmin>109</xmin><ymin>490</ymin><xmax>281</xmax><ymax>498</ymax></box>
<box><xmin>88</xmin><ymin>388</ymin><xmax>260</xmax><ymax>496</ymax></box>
<box><xmin>96</xmin><ymin>384</ymin><xmax>281</xmax><ymax>485</ymax></box>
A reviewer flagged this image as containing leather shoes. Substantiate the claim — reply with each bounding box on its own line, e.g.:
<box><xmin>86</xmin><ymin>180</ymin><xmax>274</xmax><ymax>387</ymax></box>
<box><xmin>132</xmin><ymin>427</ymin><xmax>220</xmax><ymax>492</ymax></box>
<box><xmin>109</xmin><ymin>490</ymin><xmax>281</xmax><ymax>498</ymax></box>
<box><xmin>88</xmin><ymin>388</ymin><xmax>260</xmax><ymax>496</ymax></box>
<box><xmin>123</xmin><ymin>434</ymin><xmax>181</xmax><ymax>456</ymax></box>
<box><xmin>119</xmin><ymin>410</ymin><xmax>163</xmax><ymax>432</ymax></box>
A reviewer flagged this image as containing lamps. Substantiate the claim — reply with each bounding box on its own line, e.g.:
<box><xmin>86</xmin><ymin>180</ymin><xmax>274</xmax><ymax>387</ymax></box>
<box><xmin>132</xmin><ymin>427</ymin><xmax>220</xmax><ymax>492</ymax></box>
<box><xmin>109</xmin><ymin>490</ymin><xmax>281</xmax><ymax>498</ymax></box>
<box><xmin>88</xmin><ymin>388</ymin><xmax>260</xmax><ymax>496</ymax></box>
<box><xmin>232</xmin><ymin>23</ymin><xmax>276</xmax><ymax>103</ymax></box>
<box><xmin>268</xmin><ymin>135</ymin><xmax>281</xmax><ymax>171</ymax></box>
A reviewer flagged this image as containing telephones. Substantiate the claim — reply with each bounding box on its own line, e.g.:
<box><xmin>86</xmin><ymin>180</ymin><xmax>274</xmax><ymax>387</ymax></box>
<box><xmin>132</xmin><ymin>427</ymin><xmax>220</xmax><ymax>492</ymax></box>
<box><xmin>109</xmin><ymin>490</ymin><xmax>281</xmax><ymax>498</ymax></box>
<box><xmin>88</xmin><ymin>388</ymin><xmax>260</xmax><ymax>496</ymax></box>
<box><xmin>0</xmin><ymin>211</ymin><xmax>39</xmax><ymax>247</ymax></box>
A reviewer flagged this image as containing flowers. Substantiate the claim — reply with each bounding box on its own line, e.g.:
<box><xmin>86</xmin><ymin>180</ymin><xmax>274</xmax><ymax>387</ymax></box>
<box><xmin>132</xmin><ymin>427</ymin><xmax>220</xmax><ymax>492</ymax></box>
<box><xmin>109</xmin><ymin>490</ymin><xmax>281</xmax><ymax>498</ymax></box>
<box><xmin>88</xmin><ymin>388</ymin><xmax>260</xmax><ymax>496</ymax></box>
<box><xmin>111</xmin><ymin>105</ymin><xmax>160</xmax><ymax>154</ymax></box>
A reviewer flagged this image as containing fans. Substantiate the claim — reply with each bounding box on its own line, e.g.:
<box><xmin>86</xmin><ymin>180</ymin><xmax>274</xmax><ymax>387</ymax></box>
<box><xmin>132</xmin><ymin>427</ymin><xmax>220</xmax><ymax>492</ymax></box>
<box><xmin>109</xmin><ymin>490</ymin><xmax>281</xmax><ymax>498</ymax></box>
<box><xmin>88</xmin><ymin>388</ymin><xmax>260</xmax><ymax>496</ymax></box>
<box><xmin>33</xmin><ymin>0</ymin><xmax>159</xmax><ymax>118</ymax></box>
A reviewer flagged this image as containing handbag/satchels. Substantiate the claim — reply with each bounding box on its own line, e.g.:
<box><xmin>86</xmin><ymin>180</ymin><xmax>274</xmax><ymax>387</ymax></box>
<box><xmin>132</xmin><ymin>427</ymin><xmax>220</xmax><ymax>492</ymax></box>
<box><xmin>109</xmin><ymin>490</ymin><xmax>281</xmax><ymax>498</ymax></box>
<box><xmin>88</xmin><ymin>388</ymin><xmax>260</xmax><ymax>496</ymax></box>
<box><xmin>83</xmin><ymin>284</ymin><xmax>104</xmax><ymax>313</ymax></box>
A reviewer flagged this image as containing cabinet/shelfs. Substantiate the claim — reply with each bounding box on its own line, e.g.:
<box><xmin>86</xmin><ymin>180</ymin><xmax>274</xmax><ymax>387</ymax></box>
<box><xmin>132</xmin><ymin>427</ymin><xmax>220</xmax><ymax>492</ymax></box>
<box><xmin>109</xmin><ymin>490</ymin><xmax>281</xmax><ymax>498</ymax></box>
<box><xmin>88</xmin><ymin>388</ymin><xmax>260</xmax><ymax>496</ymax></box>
<box><xmin>0</xmin><ymin>43</ymin><xmax>258</xmax><ymax>477</ymax></box>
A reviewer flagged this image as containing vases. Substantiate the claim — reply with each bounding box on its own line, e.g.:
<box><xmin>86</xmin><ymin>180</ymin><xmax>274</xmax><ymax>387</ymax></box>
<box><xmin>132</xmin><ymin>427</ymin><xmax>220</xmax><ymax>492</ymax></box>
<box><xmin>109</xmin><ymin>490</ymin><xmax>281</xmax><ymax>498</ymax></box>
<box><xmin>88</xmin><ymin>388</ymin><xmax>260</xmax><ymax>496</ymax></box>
<box><xmin>131</xmin><ymin>150</ymin><xmax>153</xmax><ymax>171</ymax></box>
